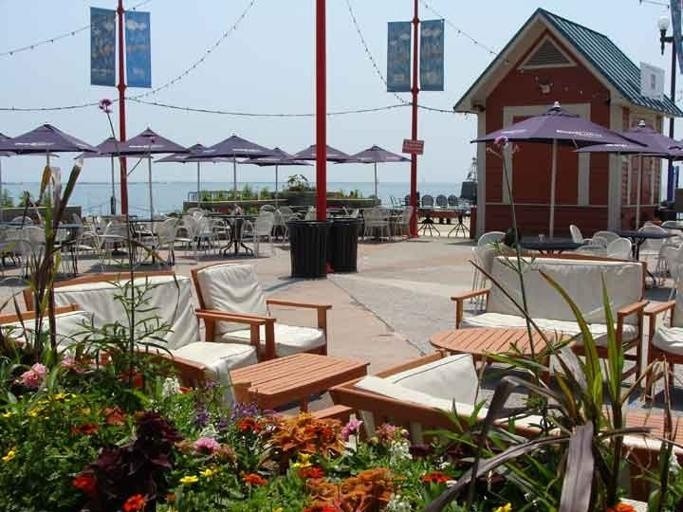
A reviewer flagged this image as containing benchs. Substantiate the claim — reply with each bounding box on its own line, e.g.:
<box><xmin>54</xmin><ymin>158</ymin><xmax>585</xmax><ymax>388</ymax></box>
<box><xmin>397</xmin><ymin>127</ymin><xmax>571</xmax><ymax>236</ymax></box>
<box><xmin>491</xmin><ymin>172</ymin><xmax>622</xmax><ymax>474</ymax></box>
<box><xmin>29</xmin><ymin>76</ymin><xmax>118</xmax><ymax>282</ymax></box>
<box><xmin>335</xmin><ymin>347</ymin><xmax>683</xmax><ymax>499</ymax></box>
<box><xmin>22</xmin><ymin>270</ymin><xmax>265</xmax><ymax>403</ymax></box>
<box><xmin>449</xmin><ymin>255</ymin><xmax>647</xmax><ymax>386</ymax></box>
<box><xmin>0</xmin><ymin>305</ymin><xmax>357</xmax><ymax>473</ymax></box>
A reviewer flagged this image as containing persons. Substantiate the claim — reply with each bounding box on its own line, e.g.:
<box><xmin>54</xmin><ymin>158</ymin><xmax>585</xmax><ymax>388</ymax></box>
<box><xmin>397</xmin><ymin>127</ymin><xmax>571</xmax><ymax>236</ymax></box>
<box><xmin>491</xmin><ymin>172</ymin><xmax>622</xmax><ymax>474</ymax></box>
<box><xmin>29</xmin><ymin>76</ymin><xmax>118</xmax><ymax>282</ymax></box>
<box><xmin>200</xmin><ymin>198</ymin><xmax>211</xmax><ymax>211</ymax></box>
<box><xmin>227</xmin><ymin>206</ymin><xmax>235</xmax><ymax>220</ymax></box>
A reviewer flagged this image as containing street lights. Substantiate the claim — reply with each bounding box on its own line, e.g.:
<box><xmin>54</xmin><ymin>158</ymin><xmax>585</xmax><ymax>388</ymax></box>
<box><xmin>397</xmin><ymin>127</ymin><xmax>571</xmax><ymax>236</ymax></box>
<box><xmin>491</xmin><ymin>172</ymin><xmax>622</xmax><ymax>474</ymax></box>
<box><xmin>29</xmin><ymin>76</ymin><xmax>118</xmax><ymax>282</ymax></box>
<box><xmin>657</xmin><ymin>16</ymin><xmax>683</xmax><ymax>202</ymax></box>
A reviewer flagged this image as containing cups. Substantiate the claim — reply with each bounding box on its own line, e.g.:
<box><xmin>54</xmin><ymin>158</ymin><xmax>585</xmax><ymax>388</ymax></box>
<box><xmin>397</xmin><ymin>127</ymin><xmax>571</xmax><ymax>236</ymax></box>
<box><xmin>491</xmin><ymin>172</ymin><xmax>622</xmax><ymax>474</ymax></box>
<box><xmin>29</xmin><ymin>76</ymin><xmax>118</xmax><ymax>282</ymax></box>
<box><xmin>538</xmin><ymin>234</ymin><xmax>544</xmax><ymax>242</ymax></box>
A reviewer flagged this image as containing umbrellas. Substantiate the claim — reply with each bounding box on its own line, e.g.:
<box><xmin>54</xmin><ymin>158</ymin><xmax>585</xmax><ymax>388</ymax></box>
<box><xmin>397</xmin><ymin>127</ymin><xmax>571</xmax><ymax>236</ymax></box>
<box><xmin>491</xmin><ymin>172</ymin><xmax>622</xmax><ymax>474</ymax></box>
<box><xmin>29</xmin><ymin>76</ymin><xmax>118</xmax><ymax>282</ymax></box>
<box><xmin>0</xmin><ymin>124</ymin><xmax>98</xmax><ymax>207</ymax></box>
<box><xmin>238</xmin><ymin>147</ymin><xmax>314</xmax><ymax>198</ymax></box>
<box><xmin>333</xmin><ymin>144</ymin><xmax>412</xmax><ymax>197</ymax></box>
<box><xmin>470</xmin><ymin>100</ymin><xmax>644</xmax><ymax>239</ymax></box>
<box><xmin>292</xmin><ymin>143</ymin><xmax>354</xmax><ymax>160</ymax></box>
<box><xmin>0</xmin><ymin>133</ymin><xmax>60</xmax><ymax>158</ymax></box>
<box><xmin>633</xmin><ymin>140</ymin><xmax>683</xmax><ymax>160</ymax></box>
<box><xmin>124</xmin><ymin>126</ymin><xmax>190</xmax><ymax>221</ymax></box>
<box><xmin>185</xmin><ymin>132</ymin><xmax>273</xmax><ymax>191</ymax></box>
<box><xmin>74</xmin><ymin>134</ymin><xmax>154</xmax><ymax>216</ymax></box>
<box><xmin>152</xmin><ymin>143</ymin><xmax>237</xmax><ymax>208</ymax></box>
<box><xmin>572</xmin><ymin>120</ymin><xmax>679</xmax><ymax>230</ymax></box>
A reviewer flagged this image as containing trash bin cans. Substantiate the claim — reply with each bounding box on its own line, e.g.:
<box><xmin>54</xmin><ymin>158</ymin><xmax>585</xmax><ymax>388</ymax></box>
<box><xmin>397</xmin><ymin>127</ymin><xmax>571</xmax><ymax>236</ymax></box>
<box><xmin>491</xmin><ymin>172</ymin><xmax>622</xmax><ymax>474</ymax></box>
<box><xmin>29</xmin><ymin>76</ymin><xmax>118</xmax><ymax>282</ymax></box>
<box><xmin>285</xmin><ymin>217</ymin><xmax>363</xmax><ymax>279</ymax></box>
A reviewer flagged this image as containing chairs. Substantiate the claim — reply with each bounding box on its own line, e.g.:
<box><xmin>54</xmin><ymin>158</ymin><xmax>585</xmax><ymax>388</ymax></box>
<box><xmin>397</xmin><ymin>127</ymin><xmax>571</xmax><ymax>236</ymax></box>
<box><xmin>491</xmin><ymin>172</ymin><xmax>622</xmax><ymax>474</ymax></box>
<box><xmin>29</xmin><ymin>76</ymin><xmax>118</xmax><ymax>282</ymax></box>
<box><xmin>639</xmin><ymin>274</ymin><xmax>683</xmax><ymax>407</ymax></box>
<box><xmin>192</xmin><ymin>261</ymin><xmax>332</xmax><ymax>358</ymax></box>
<box><xmin>472</xmin><ymin>220</ymin><xmax>683</xmax><ymax>332</ymax></box>
<box><xmin>0</xmin><ymin>194</ymin><xmax>471</xmax><ymax>278</ymax></box>
